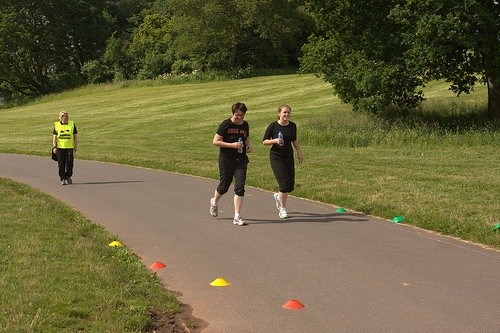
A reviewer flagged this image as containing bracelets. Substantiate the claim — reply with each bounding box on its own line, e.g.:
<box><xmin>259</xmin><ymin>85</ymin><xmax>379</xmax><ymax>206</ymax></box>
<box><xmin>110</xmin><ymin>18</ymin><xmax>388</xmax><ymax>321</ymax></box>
<box><xmin>53</xmin><ymin>146</ymin><xmax>56</xmax><ymax>149</ymax></box>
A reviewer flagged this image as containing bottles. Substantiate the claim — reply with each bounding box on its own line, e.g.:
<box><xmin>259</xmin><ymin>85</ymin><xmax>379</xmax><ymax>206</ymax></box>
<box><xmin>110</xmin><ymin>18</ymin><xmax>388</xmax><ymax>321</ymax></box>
<box><xmin>237</xmin><ymin>137</ymin><xmax>243</xmax><ymax>153</ymax></box>
<box><xmin>278</xmin><ymin>131</ymin><xmax>284</xmax><ymax>146</ymax></box>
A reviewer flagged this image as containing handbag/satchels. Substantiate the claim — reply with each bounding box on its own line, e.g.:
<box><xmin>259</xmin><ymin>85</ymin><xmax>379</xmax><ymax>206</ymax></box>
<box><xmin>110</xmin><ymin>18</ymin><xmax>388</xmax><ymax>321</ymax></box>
<box><xmin>52</xmin><ymin>147</ymin><xmax>58</xmax><ymax>161</ymax></box>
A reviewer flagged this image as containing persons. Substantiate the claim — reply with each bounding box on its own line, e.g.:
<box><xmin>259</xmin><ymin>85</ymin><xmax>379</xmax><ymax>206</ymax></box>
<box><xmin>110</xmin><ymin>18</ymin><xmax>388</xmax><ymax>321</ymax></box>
<box><xmin>52</xmin><ymin>111</ymin><xmax>78</xmax><ymax>185</ymax></box>
<box><xmin>262</xmin><ymin>104</ymin><xmax>304</xmax><ymax>219</ymax></box>
<box><xmin>209</xmin><ymin>102</ymin><xmax>251</xmax><ymax>226</ymax></box>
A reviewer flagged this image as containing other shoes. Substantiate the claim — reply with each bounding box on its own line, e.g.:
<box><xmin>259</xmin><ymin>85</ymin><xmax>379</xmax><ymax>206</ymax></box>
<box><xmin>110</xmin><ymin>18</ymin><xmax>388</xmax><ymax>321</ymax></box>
<box><xmin>67</xmin><ymin>179</ymin><xmax>72</xmax><ymax>184</ymax></box>
<box><xmin>62</xmin><ymin>180</ymin><xmax>66</xmax><ymax>185</ymax></box>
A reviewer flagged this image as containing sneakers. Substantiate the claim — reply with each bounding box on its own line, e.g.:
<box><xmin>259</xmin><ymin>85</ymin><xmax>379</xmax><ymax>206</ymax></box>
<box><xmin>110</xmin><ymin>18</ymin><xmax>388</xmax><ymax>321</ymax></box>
<box><xmin>233</xmin><ymin>217</ymin><xmax>246</xmax><ymax>226</ymax></box>
<box><xmin>210</xmin><ymin>198</ymin><xmax>217</xmax><ymax>217</ymax></box>
<box><xmin>279</xmin><ymin>209</ymin><xmax>288</xmax><ymax>218</ymax></box>
<box><xmin>273</xmin><ymin>193</ymin><xmax>281</xmax><ymax>210</ymax></box>
